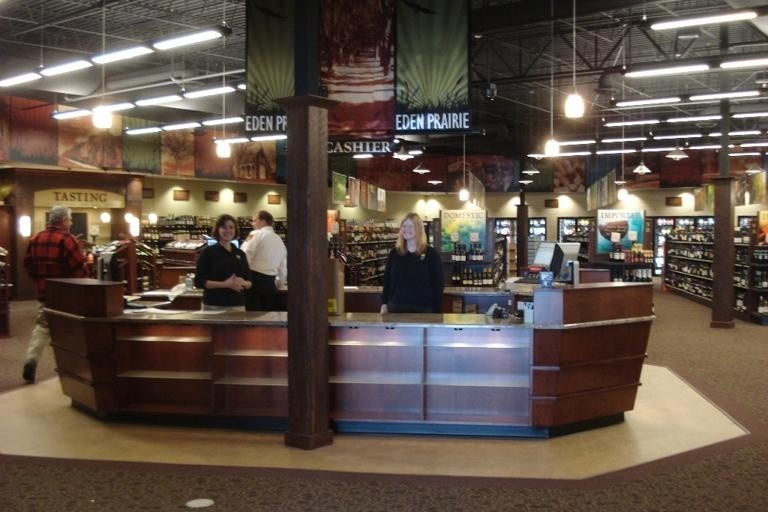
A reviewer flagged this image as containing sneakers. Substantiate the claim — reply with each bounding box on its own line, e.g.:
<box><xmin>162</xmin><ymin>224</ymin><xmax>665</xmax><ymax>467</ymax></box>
<box><xmin>23</xmin><ymin>360</ymin><xmax>36</xmax><ymax>380</ymax></box>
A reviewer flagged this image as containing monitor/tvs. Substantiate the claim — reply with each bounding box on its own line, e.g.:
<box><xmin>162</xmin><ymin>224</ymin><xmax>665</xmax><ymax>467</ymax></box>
<box><xmin>532</xmin><ymin>241</ymin><xmax>556</xmax><ymax>270</ymax></box>
<box><xmin>550</xmin><ymin>241</ymin><xmax>581</xmax><ymax>282</ymax></box>
<box><xmin>97</xmin><ymin>239</ymin><xmax>137</xmax><ymax>295</ymax></box>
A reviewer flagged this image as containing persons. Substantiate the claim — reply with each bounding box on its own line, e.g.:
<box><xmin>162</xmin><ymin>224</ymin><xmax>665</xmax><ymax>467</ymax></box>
<box><xmin>240</xmin><ymin>211</ymin><xmax>288</xmax><ymax>311</ymax></box>
<box><xmin>381</xmin><ymin>213</ymin><xmax>445</xmax><ymax>314</ymax></box>
<box><xmin>194</xmin><ymin>214</ymin><xmax>252</xmax><ymax>312</ymax></box>
<box><xmin>22</xmin><ymin>206</ymin><xmax>87</xmax><ymax>383</ymax></box>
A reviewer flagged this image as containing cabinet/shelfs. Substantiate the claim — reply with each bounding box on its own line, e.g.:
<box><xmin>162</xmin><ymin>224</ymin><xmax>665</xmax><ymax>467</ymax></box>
<box><xmin>140</xmin><ymin>209</ymin><xmax>768</xmax><ymax>327</ymax></box>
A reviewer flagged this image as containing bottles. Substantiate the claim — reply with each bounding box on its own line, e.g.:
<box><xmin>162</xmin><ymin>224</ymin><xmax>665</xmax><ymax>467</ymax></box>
<box><xmin>450</xmin><ymin>242</ymin><xmax>493</xmax><ymax>286</ymax></box>
<box><xmin>609</xmin><ymin>248</ymin><xmax>653</xmax><ymax>281</ymax></box>
<box><xmin>140</xmin><ymin>214</ymin><xmax>214</xmax><ymax>253</ymax></box>
<box><xmin>186</xmin><ymin>274</ymin><xmax>193</xmax><ymax>292</ymax></box>
<box><xmin>329</xmin><ymin>236</ymin><xmax>344</xmax><ymax>257</ymax></box>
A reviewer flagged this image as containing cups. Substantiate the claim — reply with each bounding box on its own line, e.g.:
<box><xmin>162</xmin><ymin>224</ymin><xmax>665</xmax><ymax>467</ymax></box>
<box><xmin>539</xmin><ymin>272</ymin><xmax>554</xmax><ymax>287</ymax></box>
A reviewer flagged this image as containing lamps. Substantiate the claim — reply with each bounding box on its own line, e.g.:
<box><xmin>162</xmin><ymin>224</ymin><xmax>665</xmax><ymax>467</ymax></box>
<box><xmin>51</xmin><ymin>81</ymin><xmax>287</xmax><ymax>158</ymax></box>
<box><xmin>511</xmin><ymin>1</ymin><xmax>768</xmax><ymax>208</ymax></box>
<box><xmin>1</xmin><ymin>67</ymin><xmax>42</xmax><ymax>89</ymax></box>
<box><xmin>151</xmin><ymin>21</ymin><xmax>234</xmax><ymax>53</ymax></box>
<box><xmin>40</xmin><ymin>55</ymin><xmax>95</xmax><ymax>79</ymax></box>
<box><xmin>89</xmin><ymin>41</ymin><xmax>158</xmax><ymax>66</ymax></box>
<box><xmin>353</xmin><ymin>137</ymin><xmax>487</xmax><ymax>209</ymax></box>
